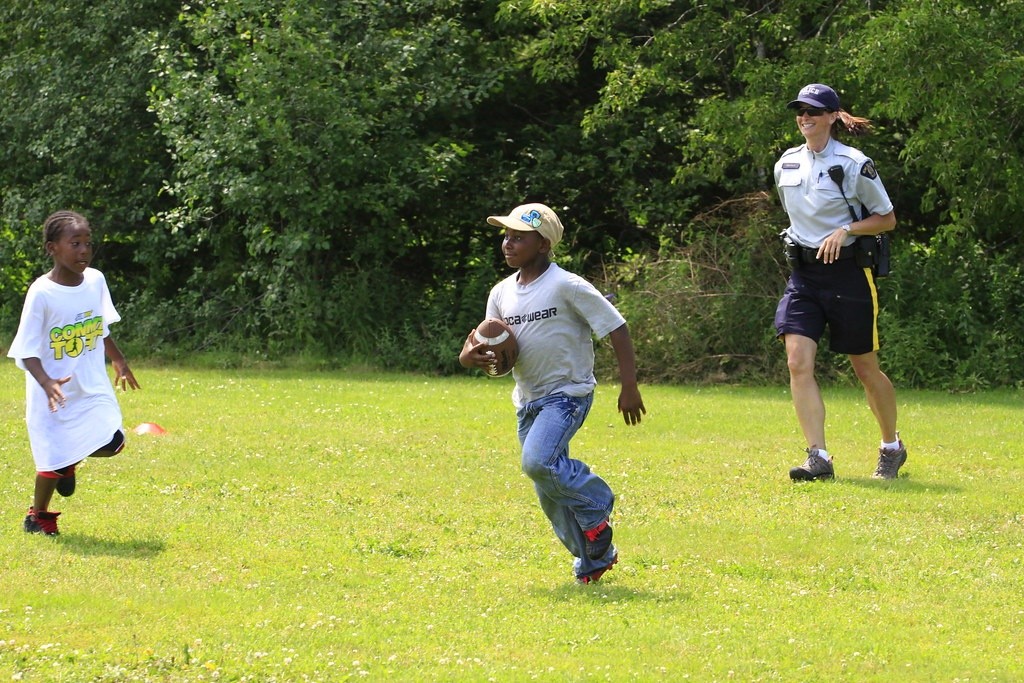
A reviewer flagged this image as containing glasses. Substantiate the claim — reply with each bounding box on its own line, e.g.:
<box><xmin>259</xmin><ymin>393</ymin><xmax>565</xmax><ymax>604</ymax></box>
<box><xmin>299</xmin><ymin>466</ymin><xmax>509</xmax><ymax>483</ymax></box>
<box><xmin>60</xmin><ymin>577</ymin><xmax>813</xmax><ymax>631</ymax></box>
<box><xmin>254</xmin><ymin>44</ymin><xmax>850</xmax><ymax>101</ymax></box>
<box><xmin>794</xmin><ymin>107</ymin><xmax>824</xmax><ymax>117</ymax></box>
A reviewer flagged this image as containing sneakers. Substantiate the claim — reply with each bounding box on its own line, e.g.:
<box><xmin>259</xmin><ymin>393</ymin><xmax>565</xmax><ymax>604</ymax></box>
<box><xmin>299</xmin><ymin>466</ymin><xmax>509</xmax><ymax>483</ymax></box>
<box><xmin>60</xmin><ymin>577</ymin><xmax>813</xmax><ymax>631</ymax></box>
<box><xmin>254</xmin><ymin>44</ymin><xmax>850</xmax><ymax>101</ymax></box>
<box><xmin>24</xmin><ymin>465</ymin><xmax>77</xmax><ymax>534</ymax></box>
<box><xmin>872</xmin><ymin>431</ymin><xmax>908</xmax><ymax>480</ymax></box>
<box><xmin>790</xmin><ymin>444</ymin><xmax>835</xmax><ymax>481</ymax></box>
<box><xmin>576</xmin><ymin>513</ymin><xmax>618</xmax><ymax>585</ymax></box>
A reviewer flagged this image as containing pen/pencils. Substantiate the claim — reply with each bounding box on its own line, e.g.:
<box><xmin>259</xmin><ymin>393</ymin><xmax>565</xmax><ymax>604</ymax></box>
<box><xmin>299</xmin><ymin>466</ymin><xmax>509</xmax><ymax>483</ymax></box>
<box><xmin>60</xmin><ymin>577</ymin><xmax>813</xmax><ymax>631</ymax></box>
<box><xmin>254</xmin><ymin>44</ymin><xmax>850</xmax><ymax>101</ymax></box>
<box><xmin>816</xmin><ymin>169</ymin><xmax>823</xmax><ymax>184</ymax></box>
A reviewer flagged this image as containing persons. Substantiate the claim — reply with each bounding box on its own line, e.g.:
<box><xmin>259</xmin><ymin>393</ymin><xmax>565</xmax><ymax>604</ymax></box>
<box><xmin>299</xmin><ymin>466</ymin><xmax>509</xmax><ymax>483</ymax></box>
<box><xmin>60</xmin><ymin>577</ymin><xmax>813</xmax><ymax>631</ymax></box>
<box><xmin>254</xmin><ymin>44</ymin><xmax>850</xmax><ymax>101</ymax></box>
<box><xmin>459</xmin><ymin>203</ymin><xmax>647</xmax><ymax>585</ymax></box>
<box><xmin>6</xmin><ymin>211</ymin><xmax>143</xmax><ymax>541</ymax></box>
<box><xmin>841</xmin><ymin>224</ymin><xmax>853</xmax><ymax>235</ymax></box>
<box><xmin>773</xmin><ymin>84</ymin><xmax>907</xmax><ymax>483</ymax></box>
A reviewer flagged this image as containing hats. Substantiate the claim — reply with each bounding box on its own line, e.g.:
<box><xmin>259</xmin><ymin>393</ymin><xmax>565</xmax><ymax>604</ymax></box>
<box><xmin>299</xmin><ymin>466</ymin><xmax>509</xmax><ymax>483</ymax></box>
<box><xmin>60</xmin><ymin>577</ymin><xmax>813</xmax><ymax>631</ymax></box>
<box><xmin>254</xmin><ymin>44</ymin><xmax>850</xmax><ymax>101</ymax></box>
<box><xmin>787</xmin><ymin>83</ymin><xmax>839</xmax><ymax>113</ymax></box>
<box><xmin>486</xmin><ymin>203</ymin><xmax>564</xmax><ymax>248</ymax></box>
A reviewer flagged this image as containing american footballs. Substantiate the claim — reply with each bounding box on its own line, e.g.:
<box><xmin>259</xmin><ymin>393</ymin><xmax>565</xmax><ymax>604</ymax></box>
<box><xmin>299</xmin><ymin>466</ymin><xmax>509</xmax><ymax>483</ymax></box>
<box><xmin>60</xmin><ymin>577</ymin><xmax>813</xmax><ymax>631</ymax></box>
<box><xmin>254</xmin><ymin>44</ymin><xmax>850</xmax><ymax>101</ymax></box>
<box><xmin>472</xmin><ymin>318</ymin><xmax>520</xmax><ymax>377</ymax></box>
